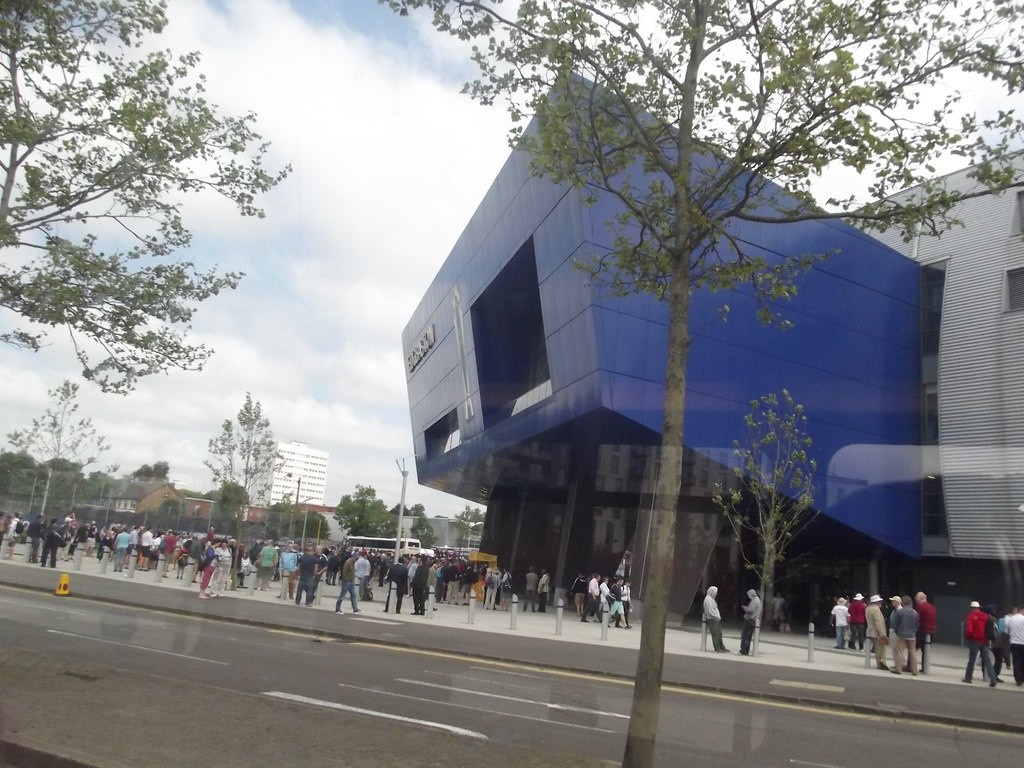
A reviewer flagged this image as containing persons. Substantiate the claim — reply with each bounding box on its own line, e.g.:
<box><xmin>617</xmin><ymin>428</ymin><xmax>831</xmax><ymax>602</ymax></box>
<box><xmin>523</xmin><ymin>566</ymin><xmax>539</xmax><ymax>612</ymax></box>
<box><xmin>0</xmin><ymin>510</ymin><xmax>512</xmax><ymax>615</ymax></box>
<box><xmin>737</xmin><ymin>589</ymin><xmax>761</xmax><ymax>655</ymax></box>
<box><xmin>829</xmin><ymin>591</ymin><xmax>937</xmax><ymax>675</ymax></box>
<box><xmin>694</xmin><ymin>581</ymin><xmax>707</xmax><ymax>618</ymax></box>
<box><xmin>962</xmin><ymin>601</ymin><xmax>1024</xmax><ymax>687</ymax></box>
<box><xmin>703</xmin><ymin>585</ymin><xmax>730</xmax><ymax>653</ymax></box>
<box><xmin>537</xmin><ymin>569</ymin><xmax>550</xmax><ymax>612</ymax></box>
<box><xmin>570</xmin><ymin>571</ymin><xmax>632</xmax><ymax>628</ymax></box>
<box><xmin>771</xmin><ymin>592</ymin><xmax>793</xmax><ymax>631</ymax></box>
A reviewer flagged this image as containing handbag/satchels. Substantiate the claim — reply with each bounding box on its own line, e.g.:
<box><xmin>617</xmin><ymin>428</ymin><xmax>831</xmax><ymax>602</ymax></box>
<box><xmin>605</xmin><ymin>584</ymin><xmax>618</xmax><ymax>604</ymax></box>
<box><xmin>502</xmin><ymin>574</ymin><xmax>514</xmax><ymax>592</ymax></box>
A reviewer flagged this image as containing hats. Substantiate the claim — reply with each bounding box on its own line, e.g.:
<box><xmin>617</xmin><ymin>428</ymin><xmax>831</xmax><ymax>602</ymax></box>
<box><xmin>971</xmin><ymin>601</ymin><xmax>980</xmax><ymax>608</ymax></box>
<box><xmin>836</xmin><ymin>598</ymin><xmax>847</xmax><ymax>605</ymax></box>
<box><xmin>870</xmin><ymin>595</ymin><xmax>884</xmax><ymax>603</ymax></box>
<box><xmin>351</xmin><ymin>551</ymin><xmax>361</xmax><ymax>559</ymax></box>
<box><xmin>889</xmin><ymin>595</ymin><xmax>902</xmax><ymax>604</ymax></box>
<box><xmin>275</xmin><ymin>545</ymin><xmax>280</xmax><ymax>550</ymax></box>
<box><xmin>64</xmin><ymin>517</ymin><xmax>73</xmax><ymax>522</ymax></box>
<box><xmin>853</xmin><ymin>593</ymin><xmax>865</xmax><ymax>601</ymax></box>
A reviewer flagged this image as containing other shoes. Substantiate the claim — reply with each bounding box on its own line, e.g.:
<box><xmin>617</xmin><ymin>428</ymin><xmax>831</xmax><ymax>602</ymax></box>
<box><xmin>410</xmin><ymin>612</ymin><xmax>425</xmax><ymax>615</ymax></box>
<box><xmin>902</xmin><ymin>665</ymin><xmax>912</xmax><ymax>672</ymax></box>
<box><xmin>962</xmin><ymin>678</ymin><xmax>973</xmax><ymax>684</ymax></box>
<box><xmin>997</xmin><ymin>677</ymin><xmax>1004</xmax><ymax>683</ymax></box>
<box><xmin>877</xmin><ymin>664</ymin><xmax>889</xmax><ymax>671</ymax></box>
<box><xmin>1016</xmin><ymin>681</ymin><xmax>1022</xmax><ymax>686</ymax></box>
<box><xmin>890</xmin><ymin>670</ymin><xmax>901</xmax><ymax>674</ymax></box>
<box><xmin>988</xmin><ymin>681</ymin><xmax>997</xmax><ymax>687</ymax></box>
<box><xmin>581</xmin><ymin>619</ymin><xmax>589</xmax><ymax>623</ymax></box>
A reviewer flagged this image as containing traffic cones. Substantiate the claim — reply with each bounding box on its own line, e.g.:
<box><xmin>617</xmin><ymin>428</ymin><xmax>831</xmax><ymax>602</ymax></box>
<box><xmin>54</xmin><ymin>573</ymin><xmax>72</xmax><ymax>596</ymax></box>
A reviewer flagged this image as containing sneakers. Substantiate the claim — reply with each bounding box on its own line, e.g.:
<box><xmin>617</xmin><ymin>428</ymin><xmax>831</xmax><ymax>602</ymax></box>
<box><xmin>335</xmin><ymin>611</ymin><xmax>344</xmax><ymax>615</ymax></box>
<box><xmin>354</xmin><ymin>608</ymin><xmax>364</xmax><ymax>614</ymax></box>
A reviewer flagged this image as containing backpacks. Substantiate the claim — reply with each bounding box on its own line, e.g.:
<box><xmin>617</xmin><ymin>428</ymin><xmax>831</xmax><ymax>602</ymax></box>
<box><xmin>14</xmin><ymin>520</ymin><xmax>25</xmax><ymax>534</ymax></box>
<box><xmin>178</xmin><ymin>554</ymin><xmax>189</xmax><ymax>567</ymax></box>
<box><xmin>63</xmin><ymin>526</ymin><xmax>72</xmax><ymax>541</ymax></box>
